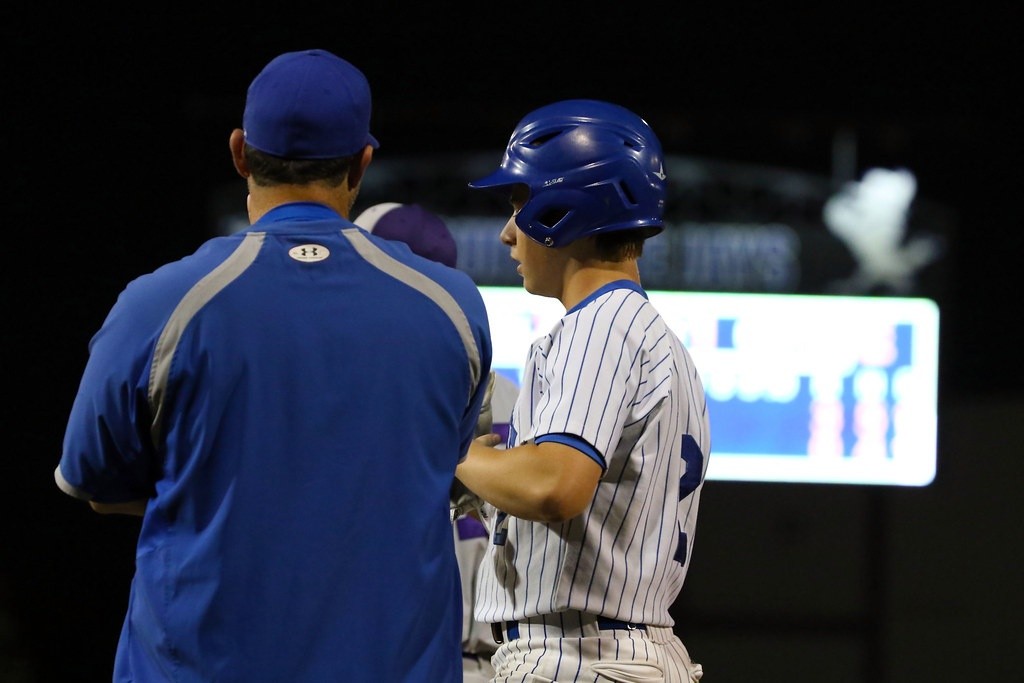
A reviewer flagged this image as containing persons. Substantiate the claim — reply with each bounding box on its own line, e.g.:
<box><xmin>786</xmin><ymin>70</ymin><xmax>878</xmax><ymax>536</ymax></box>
<box><xmin>56</xmin><ymin>51</ymin><xmax>492</xmax><ymax>683</ymax></box>
<box><xmin>453</xmin><ymin>99</ymin><xmax>709</xmax><ymax>683</ymax></box>
<box><xmin>352</xmin><ymin>199</ymin><xmax>519</xmax><ymax>683</ymax></box>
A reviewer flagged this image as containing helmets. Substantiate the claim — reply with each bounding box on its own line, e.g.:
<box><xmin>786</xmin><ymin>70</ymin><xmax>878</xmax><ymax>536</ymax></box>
<box><xmin>468</xmin><ymin>98</ymin><xmax>665</xmax><ymax>247</ymax></box>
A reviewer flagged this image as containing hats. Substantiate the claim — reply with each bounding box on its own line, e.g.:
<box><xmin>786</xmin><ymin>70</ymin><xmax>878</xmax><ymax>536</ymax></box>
<box><xmin>244</xmin><ymin>51</ymin><xmax>379</xmax><ymax>160</ymax></box>
<box><xmin>351</xmin><ymin>201</ymin><xmax>459</xmax><ymax>268</ymax></box>
